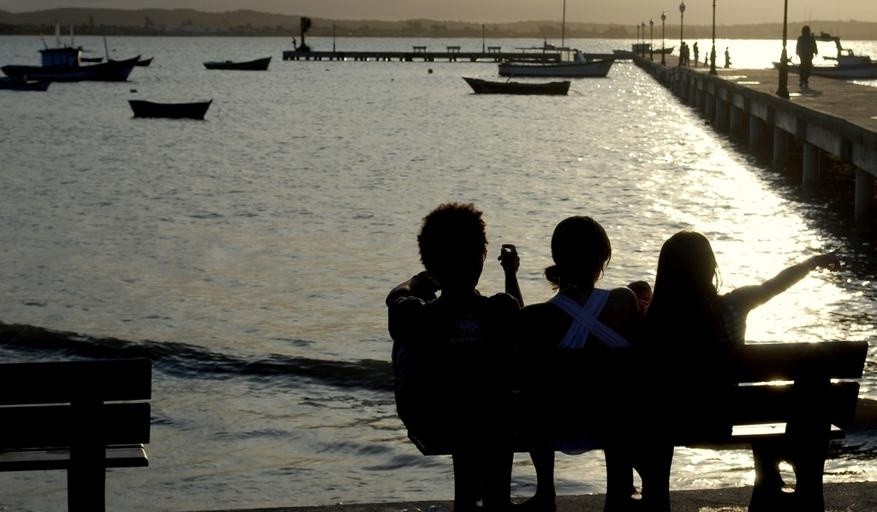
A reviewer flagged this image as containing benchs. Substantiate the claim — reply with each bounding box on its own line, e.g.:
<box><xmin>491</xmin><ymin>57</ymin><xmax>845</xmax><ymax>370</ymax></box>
<box><xmin>407</xmin><ymin>341</ymin><xmax>869</xmax><ymax>512</ymax></box>
<box><xmin>0</xmin><ymin>358</ymin><xmax>151</xmax><ymax>512</ymax></box>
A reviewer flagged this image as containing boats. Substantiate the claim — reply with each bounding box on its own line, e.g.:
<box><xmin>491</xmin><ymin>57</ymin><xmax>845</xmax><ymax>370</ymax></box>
<box><xmin>128</xmin><ymin>100</ymin><xmax>212</xmax><ymax>119</ymax></box>
<box><xmin>612</xmin><ymin>42</ymin><xmax>674</xmax><ymax>59</ymax></box>
<box><xmin>202</xmin><ymin>57</ymin><xmax>271</xmax><ymax>70</ymax></box>
<box><xmin>772</xmin><ymin>49</ymin><xmax>877</xmax><ymax>79</ymax></box>
<box><xmin>463</xmin><ymin>77</ymin><xmax>571</xmax><ymax>95</ymax></box>
<box><xmin>498</xmin><ymin>58</ymin><xmax>614</xmax><ymax>77</ymax></box>
<box><xmin>0</xmin><ymin>46</ymin><xmax>153</xmax><ymax>91</ymax></box>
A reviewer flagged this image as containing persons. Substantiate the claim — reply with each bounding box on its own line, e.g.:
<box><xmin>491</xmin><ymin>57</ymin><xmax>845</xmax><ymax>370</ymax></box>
<box><xmin>386</xmin><ymin>203</ymin><xmax>525</xmax><ymax>512</ymax></box>
<box><xmin>680</xmin><ymin>41</ymin><xmax>731</xmax><ymax>68</ymax></box>
<box><xmin>627</xmin><ymin>282</ymin><xmax>652</xmax><ymax>313</ymax></box>
<box><xmin>517</xmin><ymin>215</ymin><xmax>637</xmax><ymax>512</ymax></box>
<box><xmin>646</xmin><ymin>230</ymin><xmax>841</xmax><ymax>512</ymax></box>
<box><xmin>291</xmin><ymin>35</ymin><xmax>297</xmax><ymax>50</ymax></box>
<box><xmin>796</xmin><ymin>25</ymin><xmax>819</xmax><ymax>89</ymax></box>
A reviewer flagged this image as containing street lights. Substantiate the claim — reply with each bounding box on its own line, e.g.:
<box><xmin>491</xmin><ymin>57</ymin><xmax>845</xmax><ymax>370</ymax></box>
<box><xmin>661</xmin><ymin>11</ymin><xmax>667</xmax><ymax>64</ymax></box>
<box><xmin>636</xmin><ymin>19</ymin><xmax>654</xmax><ymax>60</ymax></box>
<box><xmin>679</xmin><ymin>0</ymin><xmax>686</xmax><ymax>42</ymax></box>
